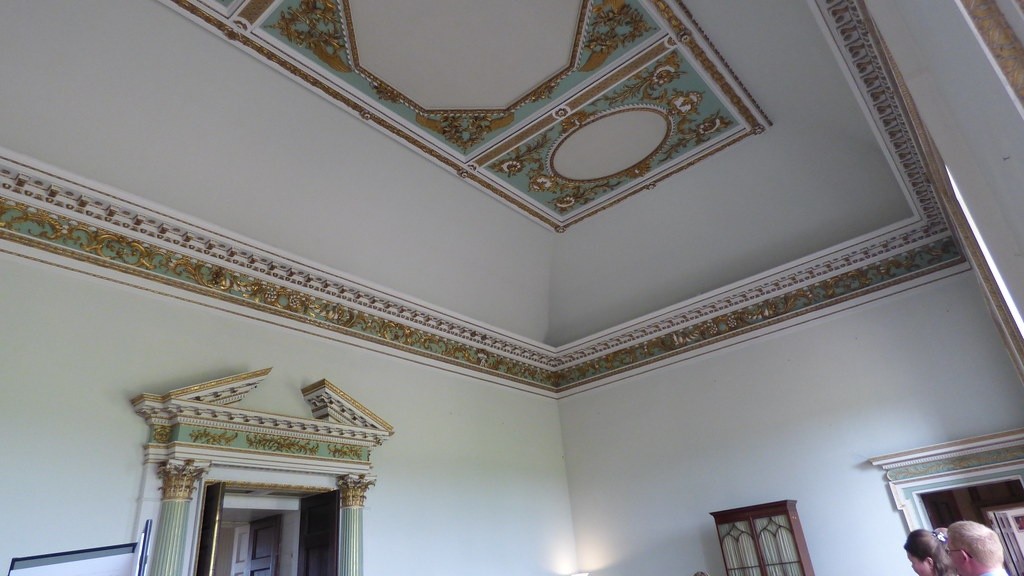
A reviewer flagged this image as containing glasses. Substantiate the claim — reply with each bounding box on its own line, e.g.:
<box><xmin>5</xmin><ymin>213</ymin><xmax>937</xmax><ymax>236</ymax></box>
<box><xmin>946</xmin><ymin>548</ymin><xmax>973</xmax><ymax>558</ymax></box>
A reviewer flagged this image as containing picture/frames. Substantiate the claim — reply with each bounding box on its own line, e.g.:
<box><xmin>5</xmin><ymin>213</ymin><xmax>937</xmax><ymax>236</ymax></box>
<box><xmin>1011</xmin><ymin>514</ymin><xmax>1024</xmax><ymax>533</ymax></box>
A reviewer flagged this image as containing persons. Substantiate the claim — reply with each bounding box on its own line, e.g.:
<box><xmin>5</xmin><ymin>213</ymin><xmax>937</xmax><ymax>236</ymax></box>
<box><xmin>949</xmin><ymin>521</ymin><xmax>1012</xmax><ymax>576</ymax></box>
<box><xmin>904</xmin><ymin>528</ymin><xmax>961</xmax><ymax>576</ymax></box>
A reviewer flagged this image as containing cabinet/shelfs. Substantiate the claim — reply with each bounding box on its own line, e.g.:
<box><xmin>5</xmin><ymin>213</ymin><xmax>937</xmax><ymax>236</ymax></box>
<box><xmin>709</xmin><ymin>499</ymin><xmax>816</xmax><ymax>576</ymax></box>
